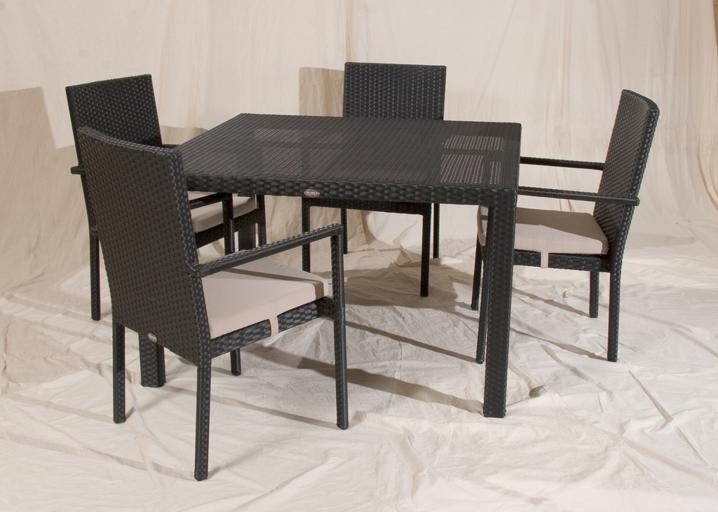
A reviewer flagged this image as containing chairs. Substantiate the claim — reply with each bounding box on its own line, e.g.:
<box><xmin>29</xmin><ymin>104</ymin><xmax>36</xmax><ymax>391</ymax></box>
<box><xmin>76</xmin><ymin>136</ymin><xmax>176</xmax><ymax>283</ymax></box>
<box><xmin>75</xmin><ymin>126</ymin><xmax>348</xmax><ymax>481</ymax></box>
<box><xmin>472</xmin><ymin>90</ymin><xmax>658</xmax><ymax>365</ymax></box>
<box><xmin>302</xmin><ymin>62</ymin><xmax>446</xmax><ymax>296</ymax></box>
<box><xmin>66</xmin><ymin>74</ymin><xmax>265</xmax><ymax>321</ymax></box>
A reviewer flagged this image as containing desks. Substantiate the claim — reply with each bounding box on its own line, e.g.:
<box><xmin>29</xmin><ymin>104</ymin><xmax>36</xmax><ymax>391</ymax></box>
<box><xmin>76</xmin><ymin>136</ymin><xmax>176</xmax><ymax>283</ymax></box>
<box><xmin>174</xmin><ymin>114</ymin><xmax>520</xmax><ymax>417</ymax></box>
